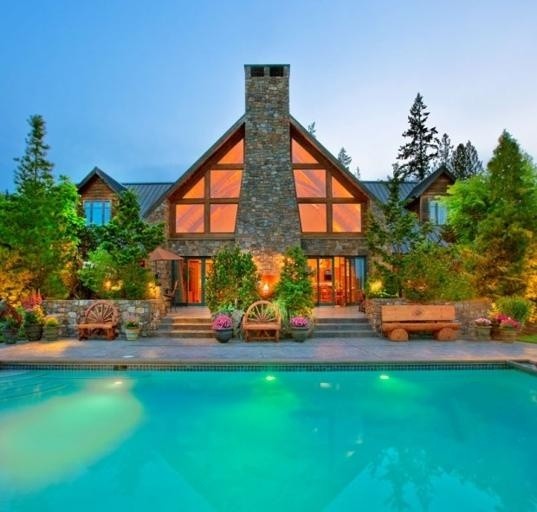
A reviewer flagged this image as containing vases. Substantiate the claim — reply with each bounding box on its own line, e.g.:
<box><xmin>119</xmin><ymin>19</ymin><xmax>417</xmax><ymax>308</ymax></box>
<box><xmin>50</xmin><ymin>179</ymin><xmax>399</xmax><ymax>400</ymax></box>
<box><xmin>292</xmin><ymin>327</ymin><xmax>309</xmax><ymax>342</ymax></box>
<box><xmin>488</xmin><ymin>327</ymin><xmax>516</xmax><ymax>343</ymax></box>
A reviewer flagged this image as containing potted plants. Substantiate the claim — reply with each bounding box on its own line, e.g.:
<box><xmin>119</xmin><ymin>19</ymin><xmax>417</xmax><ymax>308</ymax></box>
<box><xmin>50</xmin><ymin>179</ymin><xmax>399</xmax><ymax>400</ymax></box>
<box><xmin>216</xmin><ymin>330</ymin><xmax>232</xmax><ymax>343</ymax></box>
<box><xmin>124</xmin><ymin>318</ymin><xmax>141</xmax><ymax>342</ymax></box>
<box><xmin>0</xmin><ymin>289</ymin><xmax>58</xmax><ymax>344</ymax></box>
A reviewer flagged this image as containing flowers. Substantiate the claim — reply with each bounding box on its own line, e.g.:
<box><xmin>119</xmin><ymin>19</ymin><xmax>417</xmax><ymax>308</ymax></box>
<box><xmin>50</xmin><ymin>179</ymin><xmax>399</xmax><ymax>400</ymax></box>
<box><xmin>472</xmin><ymin>314</ymin><xmax>522</xmax><ymax>330</ymax></box>
<box><xmin>212</xmin><ymin>316</ymin><xmax>233</xmax><ymax>330</ymax></box>
<box><xmin>291</xmin><ymin>317</ymin><xmax>308</xmax><ymax>327</ymax></box>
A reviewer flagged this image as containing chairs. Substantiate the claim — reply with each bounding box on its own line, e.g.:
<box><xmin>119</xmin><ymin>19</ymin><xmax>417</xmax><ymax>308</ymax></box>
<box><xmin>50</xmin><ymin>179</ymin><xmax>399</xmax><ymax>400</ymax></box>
<box><xmin>240</xmin><ymin>300</ymin><xmax>282</xmax><ymax>342</ymax></box>
<box><xmin>163</xmin><ymin>279</ymin><xmax>180</xmax><ymax>313</ymax></box>
<box><xmin>79</xmin><ymin>301</ymin><xmax>118</xmax><ymax>341</ymax></box>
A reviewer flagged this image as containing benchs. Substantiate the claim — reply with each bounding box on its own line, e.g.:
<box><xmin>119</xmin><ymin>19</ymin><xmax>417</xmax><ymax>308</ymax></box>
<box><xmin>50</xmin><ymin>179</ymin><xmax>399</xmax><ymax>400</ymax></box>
<box><xmin>380</xmin><ymin>303</ymin><xmax>462</xmax><ymax>342</ymax></box>
